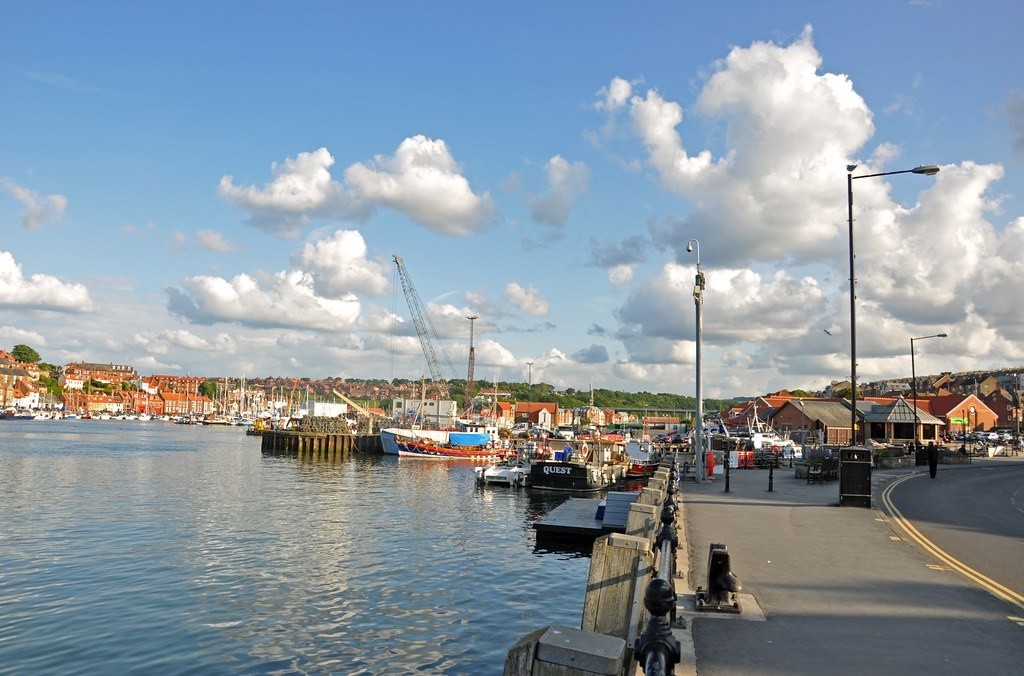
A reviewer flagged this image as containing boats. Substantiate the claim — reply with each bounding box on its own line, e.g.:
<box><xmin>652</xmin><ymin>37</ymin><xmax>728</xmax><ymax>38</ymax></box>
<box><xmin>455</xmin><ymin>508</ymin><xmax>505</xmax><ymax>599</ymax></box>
<box><xmin>377</xmin><ymin>396</ymin><xmax>807</xmax><ymax>497</ymax></box>
<box><xmin>0</xmin><ymin>378</ymin><xmax>303</xmax><ymax>436</ymax></box>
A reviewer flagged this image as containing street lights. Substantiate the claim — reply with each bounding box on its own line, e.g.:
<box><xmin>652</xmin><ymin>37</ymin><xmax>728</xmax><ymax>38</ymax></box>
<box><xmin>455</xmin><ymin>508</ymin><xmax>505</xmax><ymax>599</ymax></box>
<box><xmin>910</xmin><ymin>333</ymin><xmax>948</xmax><ymax>449</ymax></box>
<box><xmin>845</xmin><ymin>163</ymin><xmax>940</xmax><ymax>445</ymax></box>
<box><xmin>685</xmin><ymin>238</ymin><xmax>706</xmax><ymax>483</ymax></box>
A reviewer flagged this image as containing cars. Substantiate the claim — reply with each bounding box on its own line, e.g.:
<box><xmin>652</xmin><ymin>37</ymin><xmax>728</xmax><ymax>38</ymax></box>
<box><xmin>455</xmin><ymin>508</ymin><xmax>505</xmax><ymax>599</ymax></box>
<box><xmin>955</xmin><ymin>429</ymin><xmax>1024</xmax><ymax>451</ymax></box>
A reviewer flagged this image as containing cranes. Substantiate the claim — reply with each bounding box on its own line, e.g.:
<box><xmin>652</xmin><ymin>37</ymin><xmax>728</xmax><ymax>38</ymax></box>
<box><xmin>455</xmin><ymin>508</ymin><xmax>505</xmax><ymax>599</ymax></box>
<box><xmin>390</xmin><ymin>254</ymin><xmax>482</xmax><ymax>427</ymax></box>
